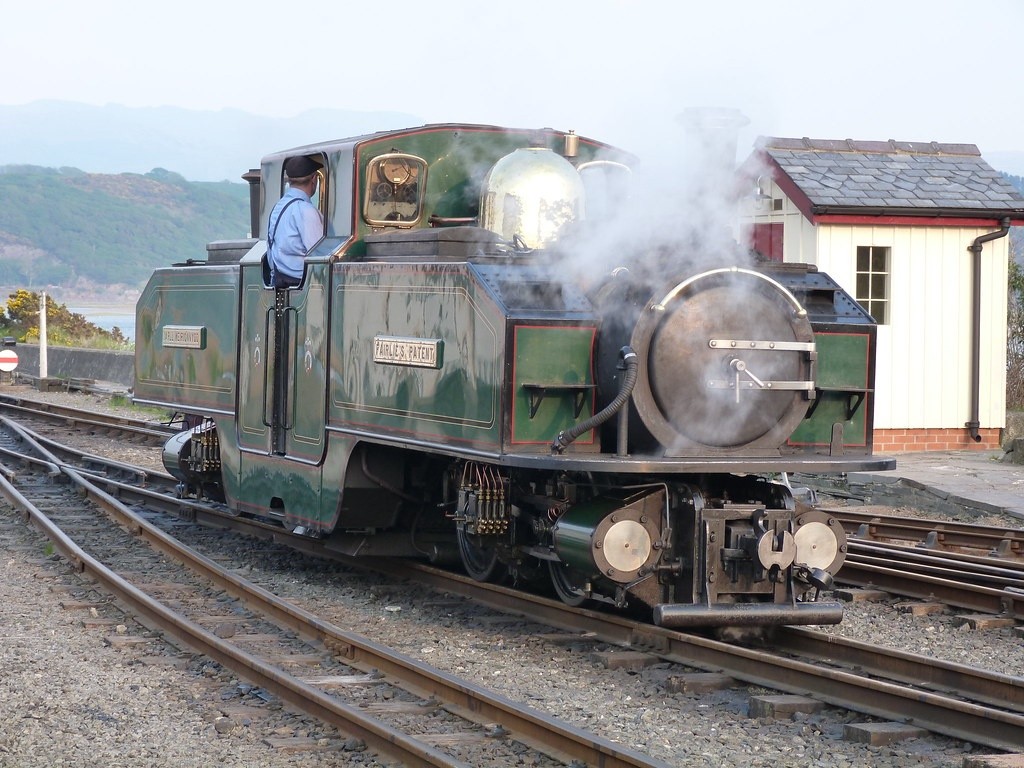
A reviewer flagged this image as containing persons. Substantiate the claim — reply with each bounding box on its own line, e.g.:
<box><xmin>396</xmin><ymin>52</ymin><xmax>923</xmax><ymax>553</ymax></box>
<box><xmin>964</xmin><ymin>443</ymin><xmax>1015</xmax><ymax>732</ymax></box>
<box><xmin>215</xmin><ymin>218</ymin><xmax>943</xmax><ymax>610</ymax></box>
<box><xmin>267</xmin><ymin>156</ymin><xmax>324</xmax><ymax>283</ymax></box>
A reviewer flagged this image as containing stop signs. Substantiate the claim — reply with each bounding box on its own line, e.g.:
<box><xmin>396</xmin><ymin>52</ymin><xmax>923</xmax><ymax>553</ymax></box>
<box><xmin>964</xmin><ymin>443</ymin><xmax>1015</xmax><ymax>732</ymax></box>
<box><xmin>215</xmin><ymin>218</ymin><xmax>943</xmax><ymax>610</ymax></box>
<box><xmin>0</xmin><ymin>349</ymin><xmax>19</xmax><ymax>371</ymax></box>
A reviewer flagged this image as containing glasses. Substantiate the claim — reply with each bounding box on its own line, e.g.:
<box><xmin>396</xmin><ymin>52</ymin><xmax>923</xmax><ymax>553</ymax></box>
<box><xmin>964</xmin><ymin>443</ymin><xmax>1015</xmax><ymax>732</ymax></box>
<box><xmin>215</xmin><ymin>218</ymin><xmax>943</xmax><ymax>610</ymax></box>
<box><xmin>316</xmin><ymin>174</ymin><xmax>323</xmax><ymax>180</ymax></box>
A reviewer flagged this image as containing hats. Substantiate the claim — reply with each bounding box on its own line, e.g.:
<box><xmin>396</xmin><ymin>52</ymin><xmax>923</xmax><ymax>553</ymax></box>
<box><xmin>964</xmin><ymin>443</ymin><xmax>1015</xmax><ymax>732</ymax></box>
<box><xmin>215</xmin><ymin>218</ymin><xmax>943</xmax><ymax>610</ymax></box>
<box><xmin>285</xmin><ymin>156</ymin><xmax>324</xmax><ymax>178</ymax></box>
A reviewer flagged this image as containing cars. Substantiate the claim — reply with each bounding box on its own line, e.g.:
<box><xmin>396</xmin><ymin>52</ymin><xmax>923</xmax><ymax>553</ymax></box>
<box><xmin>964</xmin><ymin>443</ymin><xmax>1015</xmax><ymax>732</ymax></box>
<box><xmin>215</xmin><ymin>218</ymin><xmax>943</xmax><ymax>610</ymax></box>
<box><xmin>1</xmin><ymin>337</ymin><xmax>16</xmax><ymax>347</ymax></box>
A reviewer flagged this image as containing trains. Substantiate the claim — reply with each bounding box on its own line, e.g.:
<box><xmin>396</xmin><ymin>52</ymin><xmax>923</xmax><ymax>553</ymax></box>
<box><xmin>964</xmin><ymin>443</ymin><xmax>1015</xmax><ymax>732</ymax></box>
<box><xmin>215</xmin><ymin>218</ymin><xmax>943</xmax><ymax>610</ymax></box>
<box><xmin>133</xmin><ymin>120</ymin><xmax>898</xmax><ymax>652</ymax></box>
